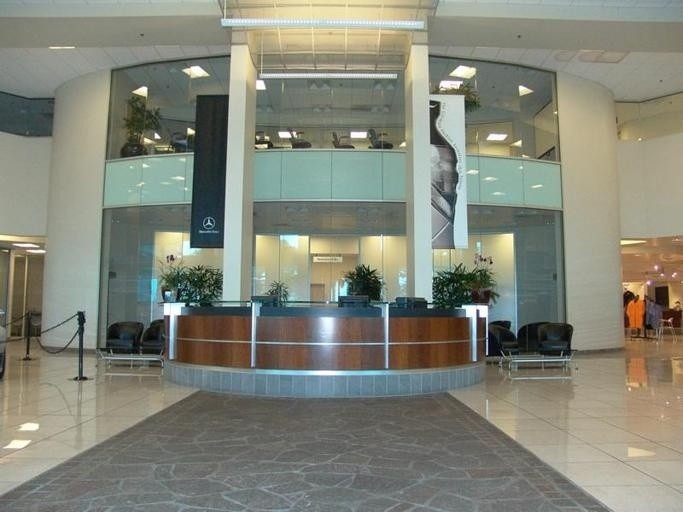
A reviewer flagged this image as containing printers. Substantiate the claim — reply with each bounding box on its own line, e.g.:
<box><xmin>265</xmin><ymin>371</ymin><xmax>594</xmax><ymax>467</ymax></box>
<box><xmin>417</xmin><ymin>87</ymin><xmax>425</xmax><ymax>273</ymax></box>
<box><xmin>395</xmin><ymin>297</ymin><xmax>426</xmax><ymax>309</ymax></box>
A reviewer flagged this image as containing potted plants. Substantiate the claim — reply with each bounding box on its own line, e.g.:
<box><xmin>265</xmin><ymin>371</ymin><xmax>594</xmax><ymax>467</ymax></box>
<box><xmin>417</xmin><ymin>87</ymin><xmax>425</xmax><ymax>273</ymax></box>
<box><xmin>469</xmin><ymin>268</ymin><xmax>496</xmax><ymax>303</ymax></box>
<box><xmin>117</xmin><ymin>95</ymin><xmax>164</xmax><ymax>158</ymax></box>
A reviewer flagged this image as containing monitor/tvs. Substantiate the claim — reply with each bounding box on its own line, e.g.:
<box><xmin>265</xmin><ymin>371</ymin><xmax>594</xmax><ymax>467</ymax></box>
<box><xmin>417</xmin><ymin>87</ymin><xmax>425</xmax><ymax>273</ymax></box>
<box><xmin>250</xmin><ymin>295</ymin><xmax>279</xmax><ymax>308</ymax></box>
<box><xmin>338</xmin><ymin>295</ymin><xmax>369</xmax><ymax>307</ymax></box>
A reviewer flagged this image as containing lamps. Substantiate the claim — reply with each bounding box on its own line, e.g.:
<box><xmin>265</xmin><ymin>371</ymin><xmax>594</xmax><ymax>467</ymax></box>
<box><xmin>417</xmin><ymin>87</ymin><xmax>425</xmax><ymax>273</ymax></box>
<box><xmin>221</xmin><ymin>0</ymin><xmax>425</xmax><ymax>30</ymax></box>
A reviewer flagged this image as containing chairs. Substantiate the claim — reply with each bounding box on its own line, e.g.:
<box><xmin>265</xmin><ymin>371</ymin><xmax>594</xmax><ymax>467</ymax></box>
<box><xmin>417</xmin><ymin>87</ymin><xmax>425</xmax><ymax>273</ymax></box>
<box><xmin>253</xmin><ymin>127</ymin><xmax>398</xmax><ymax>151</ymax></box>
<box><xmin>488</xmin><ymin>319</ymin><xmax>581</xmax><ymax>381</ymax></box>
<box><xmin>94</xmin><ymin>319</ymin><xmax>166</xmax><ymax>376</ymax></box>
<box><xmin>337</xmin><ymin>294</ymin><xmax>369</xmax><ymax>307</ymax></box>
<box><xmin>252</xmin><ymin>294</ymin><xmax>278</xmax><ymax>308</ymax></box>
